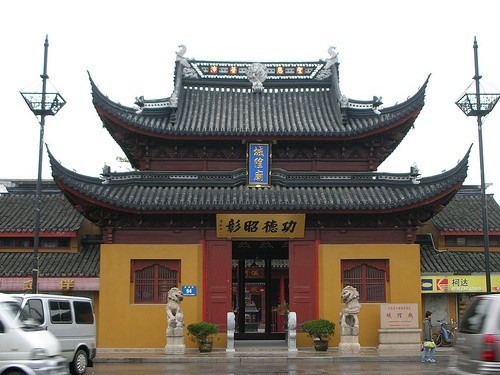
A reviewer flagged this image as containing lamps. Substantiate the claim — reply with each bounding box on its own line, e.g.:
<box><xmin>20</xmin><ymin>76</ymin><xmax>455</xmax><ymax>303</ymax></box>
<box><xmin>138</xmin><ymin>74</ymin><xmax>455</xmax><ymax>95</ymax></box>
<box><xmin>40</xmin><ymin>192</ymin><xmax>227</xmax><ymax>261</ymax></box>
<box><xmin>371</xmin><ymin>97</ymin><xmax>384</xmax><ymax>116</ymax></box>
<box><xmin>100</xmin><ymin>166</ymin><xmax>112</xmax><ymax>184</ymax></box>
<box><xmin>134</xmin><ymin>96</ymin><xmax>144</xmax><ymax>113</ymax></box>
<box><xmin>409</xmin><ymin>162</ymin><xmax>421</xmax><ymax>185</ymax></box>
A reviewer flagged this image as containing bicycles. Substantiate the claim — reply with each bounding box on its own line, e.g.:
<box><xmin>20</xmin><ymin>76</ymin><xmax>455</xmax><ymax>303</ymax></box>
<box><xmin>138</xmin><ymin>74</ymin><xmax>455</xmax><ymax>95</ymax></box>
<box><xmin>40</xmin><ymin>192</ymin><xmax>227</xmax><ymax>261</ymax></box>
<box><xmin>432</xmin><ymin>319</ymin><xmax>455</xmax><ymax>347</ymax></box>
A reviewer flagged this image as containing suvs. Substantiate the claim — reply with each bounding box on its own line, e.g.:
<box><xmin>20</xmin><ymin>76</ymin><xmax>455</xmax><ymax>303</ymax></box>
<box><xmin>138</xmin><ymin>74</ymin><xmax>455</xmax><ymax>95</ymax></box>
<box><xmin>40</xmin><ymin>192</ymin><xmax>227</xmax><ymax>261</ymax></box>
<box><xmin>451</xmin><ymin>293</ymin><xmax>500</xmax><ymax>375</ymax></box>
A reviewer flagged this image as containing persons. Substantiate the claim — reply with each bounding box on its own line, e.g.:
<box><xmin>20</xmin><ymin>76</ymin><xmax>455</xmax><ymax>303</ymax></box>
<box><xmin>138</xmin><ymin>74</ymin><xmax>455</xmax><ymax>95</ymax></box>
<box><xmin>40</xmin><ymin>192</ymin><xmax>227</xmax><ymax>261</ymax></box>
<box><xmin>421</xmin><ymin>311</ymin><xmax>436</xmax><ymax>363</ymax></box>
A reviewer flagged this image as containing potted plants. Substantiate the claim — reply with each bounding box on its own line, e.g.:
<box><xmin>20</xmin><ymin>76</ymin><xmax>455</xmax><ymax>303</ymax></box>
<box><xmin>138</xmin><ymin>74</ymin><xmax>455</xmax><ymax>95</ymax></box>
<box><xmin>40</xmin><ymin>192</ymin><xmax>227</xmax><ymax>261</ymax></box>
<box><xmin>187</xmin><ymin>322</ymin><xmax>217</xmax><ymax>354</ymax></box>
<box><xmin>300</xmin><ymin>319</ymin><xmax>335</xmax><ymax>351</ymax></box>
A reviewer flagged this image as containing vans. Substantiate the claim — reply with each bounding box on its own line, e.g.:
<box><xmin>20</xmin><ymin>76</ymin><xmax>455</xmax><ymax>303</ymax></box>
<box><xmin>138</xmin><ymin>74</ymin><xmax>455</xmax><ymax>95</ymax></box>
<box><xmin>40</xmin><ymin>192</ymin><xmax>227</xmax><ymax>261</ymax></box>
<box><xmin>9</xmin><ymin>293</ymin><xmax>98</xmax><ymax>375</ymax></box>
<box><xmin>0</xmin><ymin>292</ymin><xmax>70</xmax><ymax>375</ymax></box>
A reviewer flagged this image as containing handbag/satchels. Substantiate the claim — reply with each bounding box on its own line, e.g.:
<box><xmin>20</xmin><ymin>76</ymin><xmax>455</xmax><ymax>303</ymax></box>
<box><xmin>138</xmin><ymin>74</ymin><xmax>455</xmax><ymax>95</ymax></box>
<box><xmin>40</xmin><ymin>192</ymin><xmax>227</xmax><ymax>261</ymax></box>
<box><xmin>423</xmin><ymin>341</ymin><xmax>434</xmax><ymax>349</ymax></box>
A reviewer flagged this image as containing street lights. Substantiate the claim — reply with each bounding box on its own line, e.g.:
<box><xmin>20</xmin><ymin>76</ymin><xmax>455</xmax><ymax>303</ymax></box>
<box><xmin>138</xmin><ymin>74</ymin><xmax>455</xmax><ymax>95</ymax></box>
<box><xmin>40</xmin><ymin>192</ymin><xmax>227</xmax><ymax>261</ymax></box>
<box><xmin>18</xmin><ymin>33</ymin><xmax>67</xmax><ymax>292</ymax></box>
<box><xmin>455</xmin><ymin>36</ymin><xmax>500</xmax><ymax>295</ymax></box>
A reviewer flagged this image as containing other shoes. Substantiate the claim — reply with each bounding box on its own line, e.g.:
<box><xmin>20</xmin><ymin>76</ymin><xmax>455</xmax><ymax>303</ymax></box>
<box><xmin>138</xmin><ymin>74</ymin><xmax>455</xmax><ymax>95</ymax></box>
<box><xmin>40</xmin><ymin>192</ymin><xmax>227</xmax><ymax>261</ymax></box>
<box><xmin>428</xmin><ymin>359</ymin><xmax>435</xmax><ymax>363</ymax></box>
<box><xmin>422</xmin><ymin>359</ymin><xmax>428</xmax><ymax>362</ymax></box>
